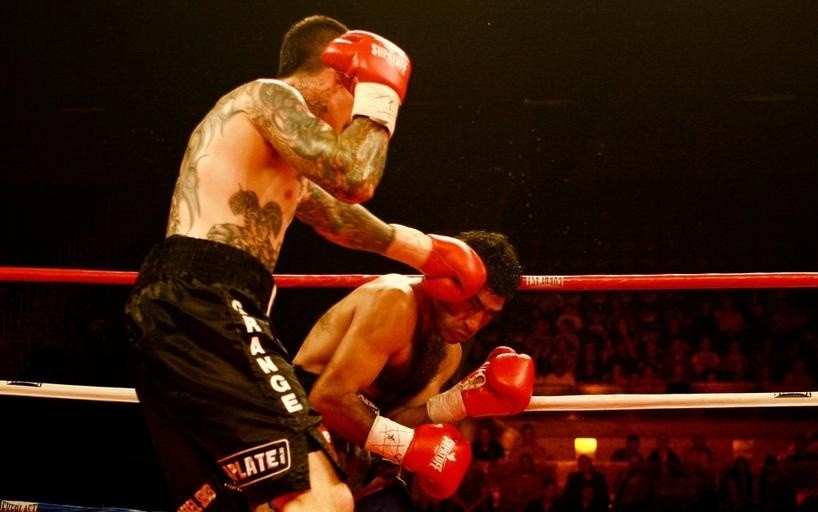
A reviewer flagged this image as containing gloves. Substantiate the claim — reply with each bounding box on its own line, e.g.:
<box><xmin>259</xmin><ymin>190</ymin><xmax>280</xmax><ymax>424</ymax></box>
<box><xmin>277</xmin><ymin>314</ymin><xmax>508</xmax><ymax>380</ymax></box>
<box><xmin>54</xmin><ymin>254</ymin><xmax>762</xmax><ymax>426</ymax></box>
<box><xmin>382</xmin><ymin>217</ymin><xmax>487</xmax><ymax>303</ymax></box>
<box><xmin>424</xmin><ymin>344</ymin><xmax>538</xmax><ymax>427</ymax></box>
<box><xmin>322</xmin><ymin>24</ymin><xmax>414</xmax><ymax>139</ymax></box>
<box><xmin>363</xmin><ymin>410</ymin><xmax>473</xmax><ymax>505</ymax></box>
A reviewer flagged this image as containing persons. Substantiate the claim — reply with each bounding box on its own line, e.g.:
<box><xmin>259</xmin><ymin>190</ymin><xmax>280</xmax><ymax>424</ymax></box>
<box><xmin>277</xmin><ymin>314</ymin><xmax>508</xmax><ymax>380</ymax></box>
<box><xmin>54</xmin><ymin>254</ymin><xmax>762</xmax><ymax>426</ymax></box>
<box><xmin>253</xmin><ymin>227</ymin><xmax>539</xmax><ymax>509</ymax></box>
<box><xmin>368</xmin><ymin>251</ymin><xmax>818</xmax><ymax>509</ymax></box>
<box><xmin>110</xmin><ymin>13</ymin><xmax>488</xmax><ymax>509</ymax></box>
